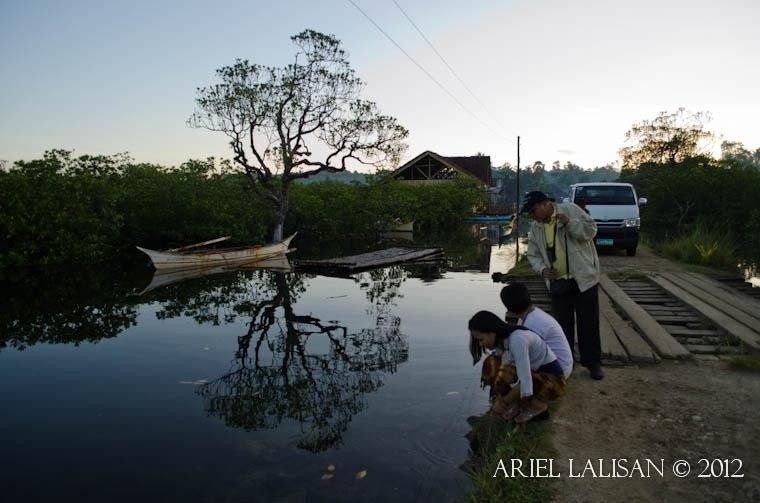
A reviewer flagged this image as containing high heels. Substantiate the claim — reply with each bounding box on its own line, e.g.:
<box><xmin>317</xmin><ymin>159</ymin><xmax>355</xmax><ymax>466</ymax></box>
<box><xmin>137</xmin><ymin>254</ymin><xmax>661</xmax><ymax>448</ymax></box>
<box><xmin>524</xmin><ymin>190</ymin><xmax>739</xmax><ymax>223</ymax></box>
<box><xmin>503</xmin><ymin>403</ymin><xmax>550</xmax><ymax>424</ymax></box>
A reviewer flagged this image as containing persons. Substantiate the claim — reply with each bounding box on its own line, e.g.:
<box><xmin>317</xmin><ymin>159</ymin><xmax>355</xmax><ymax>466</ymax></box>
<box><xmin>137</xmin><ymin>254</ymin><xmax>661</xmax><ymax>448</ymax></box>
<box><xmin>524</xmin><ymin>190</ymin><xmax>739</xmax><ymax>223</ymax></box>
<box><xmin>469</xmin><ymin>311</ymin><xmax>567</xmax><ymax>424</ymax></box>
<box><xmin>521</xmin><ymin>190</ymin><xmax>605</xmax><ymax>380</ymax></box>
<box><xmin>501</xmin><ymin>281</ymin><xmax>574</xmax><ymax>381</ymax></box>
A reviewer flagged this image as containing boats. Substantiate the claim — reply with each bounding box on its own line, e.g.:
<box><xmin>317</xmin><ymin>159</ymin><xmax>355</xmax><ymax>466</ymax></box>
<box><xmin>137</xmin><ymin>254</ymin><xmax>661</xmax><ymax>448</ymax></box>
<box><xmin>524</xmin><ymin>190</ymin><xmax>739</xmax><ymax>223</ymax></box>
<box><xmin>383</xmin><ymin>219</ymin><xmax>417</xmax><ymax>232</ymax></box>
<box><xmin>135</xmin><ymin>232</ymin><xmax>297</xmax><ymax>270</ymax></box>
<box><xmin>137</xmin><ymin>257</ymin><xmax>292</xmax><ymax>297</ymax></box>
<box><xmin>379</xmin><ymin>232</ymin><xmax>415</xmax><ymax>242</ymax></box>
<box><xmin>295</xmin><ymin>244</ymin><xmax>444</xmax><ymax>279</ymax></box>
<box><xmin>462</xmin><ymin>213</ymin><xmax>516</xmax><ymax>224</ymax></box>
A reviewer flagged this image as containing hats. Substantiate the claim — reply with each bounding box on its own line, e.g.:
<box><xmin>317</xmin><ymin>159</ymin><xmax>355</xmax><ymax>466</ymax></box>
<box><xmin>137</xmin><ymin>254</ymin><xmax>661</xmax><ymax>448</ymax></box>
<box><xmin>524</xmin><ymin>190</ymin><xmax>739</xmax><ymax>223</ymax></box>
<box><xmin>520</xmin><ymin>191</ymin><xmax>555</xmax><ymax>213</ymax></box>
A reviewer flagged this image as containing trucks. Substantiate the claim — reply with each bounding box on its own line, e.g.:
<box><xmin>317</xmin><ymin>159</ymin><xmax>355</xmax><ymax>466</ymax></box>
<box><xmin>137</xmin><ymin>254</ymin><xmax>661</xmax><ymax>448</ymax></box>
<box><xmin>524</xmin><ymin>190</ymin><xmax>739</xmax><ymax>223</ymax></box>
<box><xmin>565</xmin><ymin>182</ymin><xmax>648</xmax><ymax>255</ymax></box>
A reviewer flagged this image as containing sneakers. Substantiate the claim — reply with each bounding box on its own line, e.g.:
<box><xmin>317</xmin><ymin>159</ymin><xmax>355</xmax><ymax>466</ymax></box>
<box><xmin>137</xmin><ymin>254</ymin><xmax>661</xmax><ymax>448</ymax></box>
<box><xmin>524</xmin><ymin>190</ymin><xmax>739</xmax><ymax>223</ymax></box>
<box><xmin>587</xmin><ymin>364</ymin><xmax>603</xmax><ymax>379</ymax></box>
